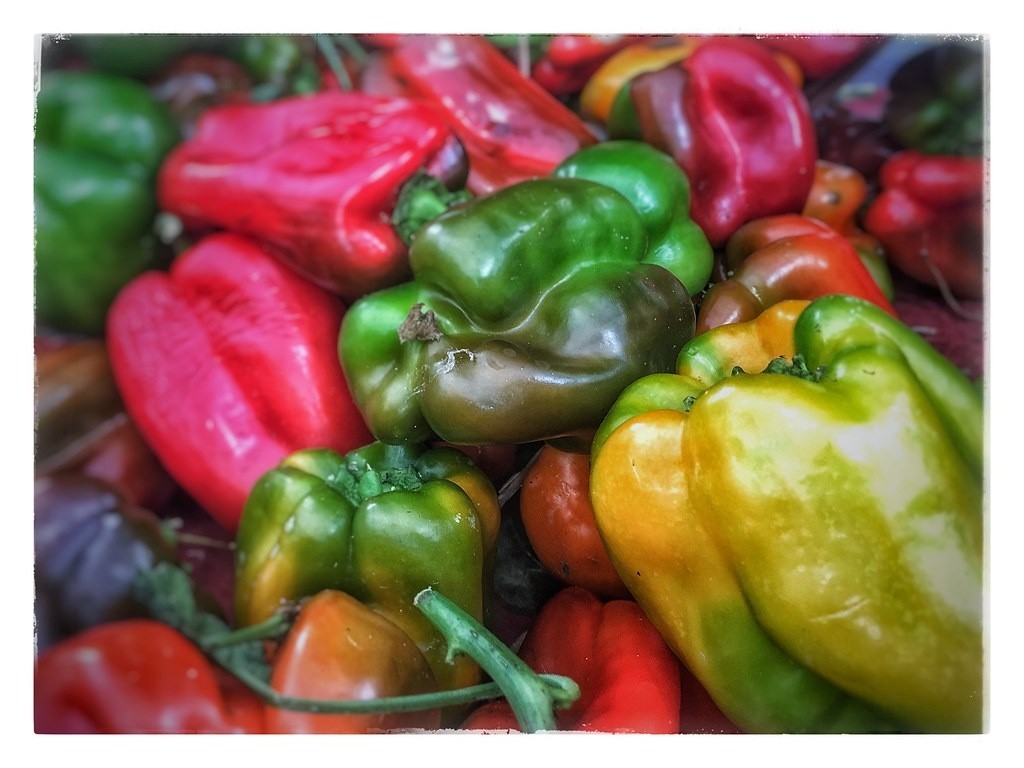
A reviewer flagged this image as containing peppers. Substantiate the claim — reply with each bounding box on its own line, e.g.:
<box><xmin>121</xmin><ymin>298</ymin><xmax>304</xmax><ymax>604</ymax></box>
<box><xmin>34</xmin><ymin>31</ymin><xmax>985</xmax><ymax>733</ymax></box>
